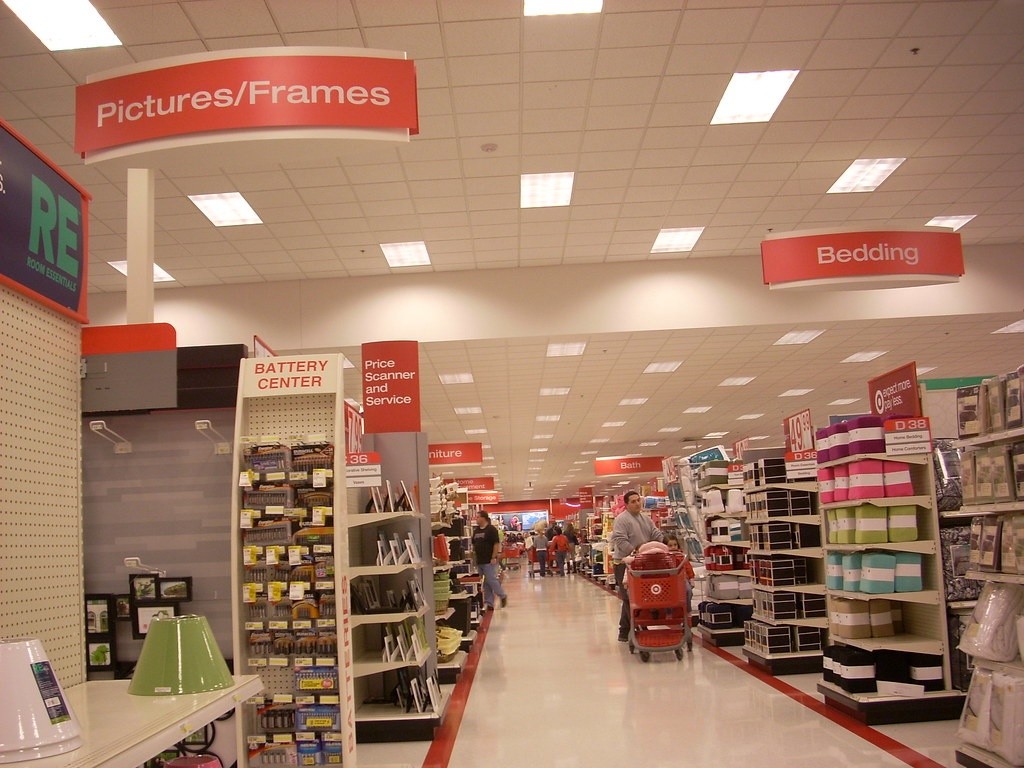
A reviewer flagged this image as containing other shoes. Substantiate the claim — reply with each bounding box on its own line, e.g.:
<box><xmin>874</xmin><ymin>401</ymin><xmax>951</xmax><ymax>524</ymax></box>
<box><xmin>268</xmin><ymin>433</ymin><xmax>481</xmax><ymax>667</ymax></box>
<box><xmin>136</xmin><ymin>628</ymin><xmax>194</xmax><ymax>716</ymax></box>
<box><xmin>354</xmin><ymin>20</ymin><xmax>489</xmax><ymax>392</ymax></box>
<box><xmin>500</xmin><ymin>594</ymin><xmax>507</xmax><ymax>608</ymax></box>
<box><xmin>618</xmin><ymin>632</ymin><xmax>627</xmax><ymax>641</ymax></box>
<box><xmin>488</xmin><ymin>605</ymin><xmax>494</xmax><ymax>611</ymax></box>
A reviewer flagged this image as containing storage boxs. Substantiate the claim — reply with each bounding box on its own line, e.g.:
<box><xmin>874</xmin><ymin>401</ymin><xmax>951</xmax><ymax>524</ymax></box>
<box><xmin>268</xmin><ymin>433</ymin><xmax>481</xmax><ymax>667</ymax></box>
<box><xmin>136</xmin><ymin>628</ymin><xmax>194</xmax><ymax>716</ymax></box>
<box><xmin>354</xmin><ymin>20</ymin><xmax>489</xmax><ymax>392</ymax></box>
<box><xmin>701</xmin><ymin>459</ymin><xmax>826</xmax><ymax>655</ymax></box>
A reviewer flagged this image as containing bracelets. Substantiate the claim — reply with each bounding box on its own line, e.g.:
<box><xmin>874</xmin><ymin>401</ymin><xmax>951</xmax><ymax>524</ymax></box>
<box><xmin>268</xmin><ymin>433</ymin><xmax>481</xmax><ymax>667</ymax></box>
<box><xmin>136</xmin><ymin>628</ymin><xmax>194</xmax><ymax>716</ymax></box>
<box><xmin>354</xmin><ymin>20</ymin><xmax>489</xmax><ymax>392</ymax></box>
<box><xmin>491</xmin><ymin>557</ymin><xmax>497</xmax><ymax>559</ymax></box>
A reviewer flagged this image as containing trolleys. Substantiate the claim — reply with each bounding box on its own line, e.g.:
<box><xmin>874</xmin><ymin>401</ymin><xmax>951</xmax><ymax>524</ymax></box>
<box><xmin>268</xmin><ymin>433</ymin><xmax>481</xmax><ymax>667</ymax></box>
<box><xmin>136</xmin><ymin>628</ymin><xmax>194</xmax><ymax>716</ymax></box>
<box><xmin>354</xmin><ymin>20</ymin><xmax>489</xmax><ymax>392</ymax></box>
<box><xmin>546</xmin><ymin>541</ymin><xmax>563</xmax><ymax>577</ymax></box>
<box><xmin>623</xmin><ymin>553</ymin><xmax>694</xmax><ymax>662</ymax></box>
<box><xmin>526</xmin><ymin>544</ymin><xmax>548</xmax><ymax>577</ymax></box>
<box><xmin>505</xmin><ymin>548</ymin><xmax>521</xmax><ymax>570</ymax></box>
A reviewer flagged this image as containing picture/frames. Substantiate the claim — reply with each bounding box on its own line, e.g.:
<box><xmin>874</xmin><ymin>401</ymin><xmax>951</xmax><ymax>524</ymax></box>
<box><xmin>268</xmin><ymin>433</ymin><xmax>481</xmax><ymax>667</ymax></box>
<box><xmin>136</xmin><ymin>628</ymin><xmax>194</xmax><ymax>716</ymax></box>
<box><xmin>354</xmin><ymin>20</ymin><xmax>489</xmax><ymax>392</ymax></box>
<box><xmin>350</xmin><ymin>478</ymin><xmax>443</xmax><ymax>713</ymax></box>
<box><xmin>85</xmin><ymin>573</ymin><xmax>195</xmax><ymax>675</ymax></box>
<box><xmin>143</xmin><ymin>708</ymin><xmax>236</xmax><ymax>768</ymax></box>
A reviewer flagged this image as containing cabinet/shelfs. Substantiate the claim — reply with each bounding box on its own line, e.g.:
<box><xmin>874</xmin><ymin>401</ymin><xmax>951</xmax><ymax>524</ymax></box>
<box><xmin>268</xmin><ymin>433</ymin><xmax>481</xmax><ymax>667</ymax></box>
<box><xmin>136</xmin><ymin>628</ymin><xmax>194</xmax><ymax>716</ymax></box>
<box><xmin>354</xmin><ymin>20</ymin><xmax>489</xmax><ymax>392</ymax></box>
<box><xmin>430</xmin><ymin>478</ymin><xmax>488</xmax><ymax>683</ymax></box>
<box><xmin>816</xmin><ymin>426</ymin><xmax>1024</xmax><ymax>768</ymax></box>
<box><xmin>349</xmin><ymin>512</ymin><xmax>449</xmax><ymax>723</ymax></box>
<box><xmin>697</xmin><ymin>483</ymin><xmax>752</xmax><ymax>648</ymax></box>
<box><xmin>574</xmin><ymin>455</ymin><xmax>704</xmax><ymax>629</ymax></box>
<box><xmin>742</xmin><ymin>482</ymin><xmax>830</xmax><ymax>676</ymax></box>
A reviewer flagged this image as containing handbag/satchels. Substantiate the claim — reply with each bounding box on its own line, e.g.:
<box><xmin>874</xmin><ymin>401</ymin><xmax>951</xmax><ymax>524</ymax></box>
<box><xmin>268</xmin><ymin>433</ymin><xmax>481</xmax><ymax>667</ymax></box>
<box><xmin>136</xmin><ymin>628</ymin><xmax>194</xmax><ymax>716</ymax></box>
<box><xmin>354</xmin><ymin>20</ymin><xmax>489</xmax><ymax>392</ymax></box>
<box><xmin>564</xmin><ymin>537</ymin><xmax>569</xmax><ymax>550</ymax></box>
<box><xmin>573</xmin><ymin>534</ymin><xmax>579</xmax><ymax>545</ymax></box>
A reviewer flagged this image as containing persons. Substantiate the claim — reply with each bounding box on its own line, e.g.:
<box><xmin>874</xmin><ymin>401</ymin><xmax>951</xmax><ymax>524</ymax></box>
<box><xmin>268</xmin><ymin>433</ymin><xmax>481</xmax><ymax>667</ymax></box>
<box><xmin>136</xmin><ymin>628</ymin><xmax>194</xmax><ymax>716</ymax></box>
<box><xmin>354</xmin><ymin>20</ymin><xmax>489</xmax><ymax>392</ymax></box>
<box><xmin>497</xmin><ymin>515</ymin><xmax>508</xmax><ymax>531</ymax></box>
<box><xmin>503</xmin><ymin>532</ymin><xmax>533</xmax><ymax>570</ymax></box>
<box><xmin>534</xmin><ymin>522</ymin><xmax>579</xmax><ymax>577</ymax></box>
<box><xmin>662</xmin><ymin>535</ymin><xmax>695</xmax><ymax>616</ymax></box>
<box><xmin>471</xmin><ymin>510</ymin><xmax>507</xmax><ymax>611</ymax></box>
<box><xmin>612</xmin><ymin>491</ymin><xmax>665</xmax><ymax>644</ymax></box>
<box><xmin>510</xmin><ymin>516</ymin><xmax>521</xmax><ymax>532</ymax></box>
